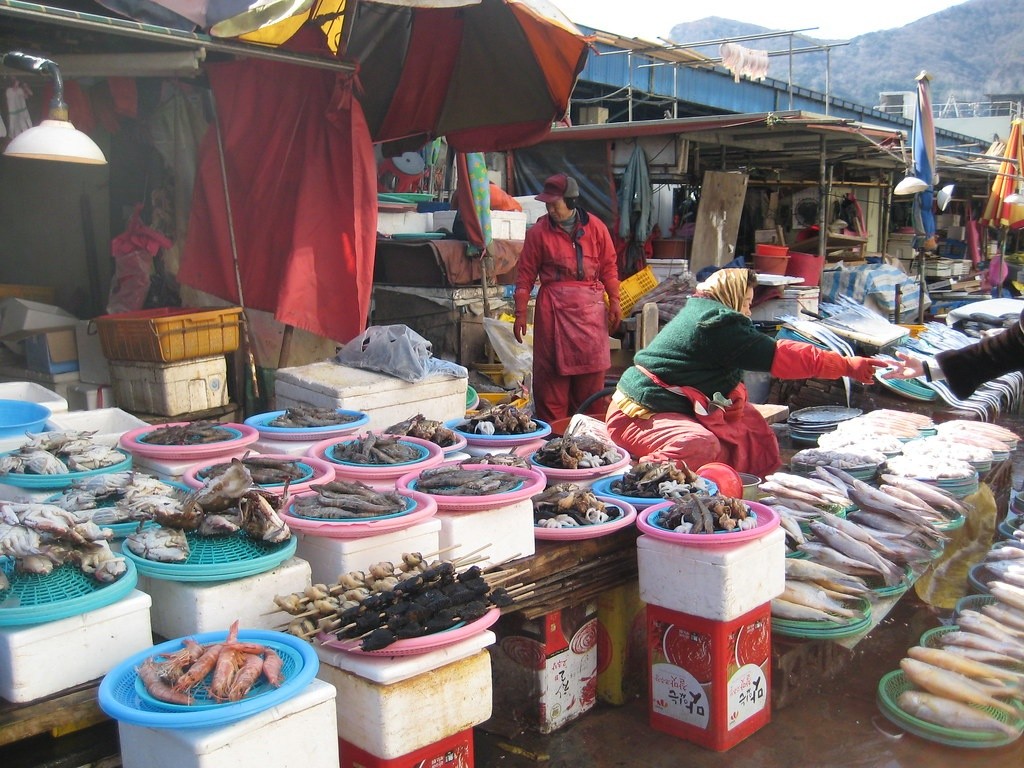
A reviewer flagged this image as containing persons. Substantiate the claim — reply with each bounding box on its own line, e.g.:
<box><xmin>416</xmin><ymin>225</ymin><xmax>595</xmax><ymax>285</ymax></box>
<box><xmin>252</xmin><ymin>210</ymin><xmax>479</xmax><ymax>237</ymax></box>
<box><xmin>880</xmin><ymin>307</ymin><xmax>1023</xmax><ymax>401</ymax></box>
<box><xmin>446</xmin><ymin>174</ymin><xmax>522</xmax><ymax>240</ymax></box>
<box><xmin>510</xmin><ymin>174</ymin><xmax>623</xmax><ymax>426</ymax></box>
<box><xmin>604</xmin><ymin>267</ymin><xmax>890</xmax><ymax>473</ymax></box>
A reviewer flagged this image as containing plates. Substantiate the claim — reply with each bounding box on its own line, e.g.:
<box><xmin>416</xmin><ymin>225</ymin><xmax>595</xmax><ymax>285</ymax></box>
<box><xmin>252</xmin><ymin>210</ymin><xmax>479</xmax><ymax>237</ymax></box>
<box><xmin>787</xmin><ymin>405</ymin><xmax>863</xmax><ymax>448</ymax></box>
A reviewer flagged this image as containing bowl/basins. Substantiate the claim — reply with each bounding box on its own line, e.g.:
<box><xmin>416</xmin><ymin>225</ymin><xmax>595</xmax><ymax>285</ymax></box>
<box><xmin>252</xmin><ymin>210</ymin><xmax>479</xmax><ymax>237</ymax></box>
<box><xmin>0</xmin><ymin>399</ymin><xmax>53</xmax><ymax>439</ymax></box>
<box><xmin>697</xmin><ymin>462</ymin><xmax>743</xmax><ymax>500</ymax></box>
<box><xmin>652</xmin><ymin>239</ymin><xmax>690</xmax><ymax>259</ymax></box>
<box><xmin>737</xmin><ymin>472</ymin><xmax>762</xmax><ymax>501</ymax></box>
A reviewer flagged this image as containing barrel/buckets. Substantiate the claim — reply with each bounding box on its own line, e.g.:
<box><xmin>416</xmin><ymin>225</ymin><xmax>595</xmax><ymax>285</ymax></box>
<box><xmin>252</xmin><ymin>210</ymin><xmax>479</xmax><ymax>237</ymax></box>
<box><xmin>752</xmin><ymin>245</ymin><xmax>824</xmax><ymax>288</ymax></box>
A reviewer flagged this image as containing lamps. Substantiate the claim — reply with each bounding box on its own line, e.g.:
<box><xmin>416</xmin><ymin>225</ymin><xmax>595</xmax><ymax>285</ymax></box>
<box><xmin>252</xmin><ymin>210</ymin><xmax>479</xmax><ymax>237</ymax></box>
<box><xmin>895</xmin><ymin>133</ymin><xmax>928</xmax><ymax>196</ymax></box>
<box><xmin>0</xmin><ymin>46</ymin><xmax>110</xmax><ymax>167</ymax></box>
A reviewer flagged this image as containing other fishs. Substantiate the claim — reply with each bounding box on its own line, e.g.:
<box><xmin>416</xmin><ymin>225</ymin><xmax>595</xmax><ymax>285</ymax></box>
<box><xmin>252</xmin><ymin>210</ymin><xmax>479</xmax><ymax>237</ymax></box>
<box><xmin>872</xmin><ymin>321</ymin><xmax>1024</xmax><ymax>423</ymax></box>
<box><xmin>897</xmin><ymin>530</ymin><xmax>1024</xmax><ymax>737</ymax></box>
<box><xmin>762</xmin><ymin>467</ymin><xmax>964</xmax><ymax>620</ymax></box>
<box><xmin>774</xmin><ymin>292</ymin><xmax>899</xmax><ymax>408</ymax></box>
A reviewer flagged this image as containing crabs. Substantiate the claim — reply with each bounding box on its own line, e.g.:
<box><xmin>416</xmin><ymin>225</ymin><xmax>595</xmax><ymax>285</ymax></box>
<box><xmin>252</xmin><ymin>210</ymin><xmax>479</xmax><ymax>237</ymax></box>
<box><xmin>463</xmin><ymin>404</ymin><xmax>536</xmax><ymax>435</ymax></box>
<box><xmin>0</xmin><ymin>428</ymin><xmax>127</xmax><ymax>476</ymax></box>
<box><xmin>0</xmin><ymin>501</ymin><xmax>126</xmax><ymax>584</ymax></box>
<box><xmin>386</xmin><ymin>413</ymin><xmax>457</xmax><ymax>448</ymax></box>
<box><xmin>56</xmin><ymin>458</ymin><xmax>291</xmax><ymax>561</ymax></box>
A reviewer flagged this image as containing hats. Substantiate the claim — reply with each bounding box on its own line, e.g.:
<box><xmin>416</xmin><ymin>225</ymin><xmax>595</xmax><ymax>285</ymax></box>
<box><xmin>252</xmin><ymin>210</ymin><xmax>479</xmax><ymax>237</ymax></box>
<box><xmin>534</xmin><ymin>173</ymin><xmax>579</xmax><ymax>202</ymax></box>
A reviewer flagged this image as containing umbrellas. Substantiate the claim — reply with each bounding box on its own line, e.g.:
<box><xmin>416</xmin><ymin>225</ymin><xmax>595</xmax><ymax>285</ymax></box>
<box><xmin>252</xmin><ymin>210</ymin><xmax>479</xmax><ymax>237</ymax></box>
<box><xmin>910</xmin><ymin>69</ymin><xmax>941</xmax><ymax>321</ymax></box>
<box><xmin>210</xmin><ymin>0</ymin><xmax>604</xmax><ymax>152</ymax></box>
<box><xmin>976</xmin><ymin>118</ymin><xmax>1024</xmax><ymax>298</ymax></box>
<box><xmin>452</xmin><ymin>149</ymin><xmax>498</xmax><ymax>317</ymax></box>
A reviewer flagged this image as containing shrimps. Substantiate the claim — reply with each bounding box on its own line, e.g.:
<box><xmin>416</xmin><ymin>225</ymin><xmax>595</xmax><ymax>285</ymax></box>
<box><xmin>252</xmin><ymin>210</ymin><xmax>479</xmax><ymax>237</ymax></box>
<box><xmin>536</xmin><ymin>434</ymin><xmax>613</xmax><ymax>469</ymax></box>
<box><xmin>139</xmin><ymin>619</ymin><xmax>283</xmax><ymax>705</ymax></box>
<box><xmin>294</xmin><ymin>480</ymin><xmax>408</xmax><ymax>517</ymax></box>
<box><xmin>198</xmin><ymin>450</ymin><xmax>305</xmax><ymax>484</ymax></box>
<box><xmin>332</xmin><ymin>431</ymin><xmax>422</xmax><ymax>466</ymax></box>
<box><xmin>412</xmin><ymin>463</ymin><xmax>525</xmax><ymax>496</ymax></box>
<box><xmin>532</xmin><ymin>482</ymin><xmax>608</xmax><ymax>527</ymax></box>
<box><xmin>141</xmin><ymin>418</ymin><xmax>232</xmax><ymax>445</ymax></box>
<box><xmin>268</xmin><ymin>404</ymin><xmax>361</xmax><ymax>428</ymax></box>
<box><xmin>611</xmin><ymin>459</ymin><xmax>698</xmax><ymax>498</ymax></box>
<box><xmin>655</xmin><ymin>491</ymin><xmax>750</xmax><ymax>536</ymax></box>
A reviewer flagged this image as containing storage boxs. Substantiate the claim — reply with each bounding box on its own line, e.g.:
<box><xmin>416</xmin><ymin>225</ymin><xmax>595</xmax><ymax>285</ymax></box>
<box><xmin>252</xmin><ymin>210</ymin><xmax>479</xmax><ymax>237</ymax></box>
<box><xmin>0</xmin><ymin>589</ymin><xmax>155</xmax><ymax>704</ymax></box>
<box><xmin>377</xmin><ymin>194</ymin><xmax>548</xmax><ymax>241</ymax></box>
<box><xmin>580</xmin><ymin>104</ymin><xmax>610</xmax><ymax>124</ymax></box>
<box><xmin>118</xmin><ymin>677</ymin><xmax>338</xmax><ymax>768</ymax></box>
<box><xmin>135</xmin><ymin>557</ymin><xmax>313</xmax><ymax>640</ymax></box>
<box><xmin>275</xmin><ymin>358</ymin><xmax>467</xmax><ymax>428</ymax></box>
<box><xmin>92</xmin><ymin>305</ymin><xmax>243</xmax><ymax>362</ymax></box>
<box><xmin>636</xmin><ymin>526</ymin><xmax>786</xmax><ymax>754</ymax></box>
<box><xmin>107</xmin><ymin>355</ymin><xmax>228</xmax><ymax>416</ymax></box>
<box><xmin>886</xmin><ymin>211</ymin><xmax>974</xmax><ymax>276</ymax></box>
<box><xmin>0</xmin><ymin>283</ymin><xmax>111</xmax><ymax>386</ymax></box>
<box><xmin>306</xmin><ymin>639</ymin><xmax>493</xmax><ymax>768</ymax></box>
<box><xmin>437</xmin><ymin>499</ymin><xmax>535</xmax><ymax>569</ymax></box>
<box><xmin>748</xmin><ymin>272</ymin><xmax>820</xmax><ymax>322</ymax></box>
<box><xmin>491</xmin><ymin>598</ymin><xmax>598</xmax><ymax>734</ymax></box>
<box><xmin>293</xmin><ymin>517</ymin><xmax>442</xmax><ymax>587</ymax></box>
<box><xmin>602</xmin><ymin>258</ymin><xmax>689</xmax><ymax>333</ymax></box>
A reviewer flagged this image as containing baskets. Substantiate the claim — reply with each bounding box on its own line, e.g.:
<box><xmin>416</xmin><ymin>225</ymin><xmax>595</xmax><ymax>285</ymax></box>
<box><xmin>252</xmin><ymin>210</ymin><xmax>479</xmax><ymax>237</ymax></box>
<box><xmin>466</xmin><ymin>392</ymin><xmax>530</xmax><ymax>417</ymax></box>
<box><xmin>606</xmin><ymin>265</ymin><xmax>657</xmax><ymax>328</ymax></box>
<box><xmin>0</xmin><ymin>329</ymin><xmax>1024</xmax><ymax>748</ymax></box>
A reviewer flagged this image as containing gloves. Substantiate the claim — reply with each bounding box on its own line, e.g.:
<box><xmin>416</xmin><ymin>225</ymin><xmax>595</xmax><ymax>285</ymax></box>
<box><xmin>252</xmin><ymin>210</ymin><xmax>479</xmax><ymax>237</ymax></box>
<box><xmin>513</xmin><ymin>293</ymin><xmax>529</xmax><ymax>342</ymax></box>
<box><xmin>772</xmin><ymin>338</ymin><xmax>889</xmax><ymax>384</ymax></box>
<box><xmin>722</xmin><ymin>382</ymin><xmax>748</xmax><ymax>424</ymax></box>
<box><xmin>604</xmin><ymin>279</ymin><xmax>621</xmax><ymax>331</ymax></box>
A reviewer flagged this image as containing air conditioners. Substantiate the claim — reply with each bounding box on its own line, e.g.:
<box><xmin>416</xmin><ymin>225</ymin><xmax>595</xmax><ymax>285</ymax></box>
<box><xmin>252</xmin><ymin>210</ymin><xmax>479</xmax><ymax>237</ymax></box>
<box><xmin>879</xmin><ymin>92</ymin><xmax>918</xmax><ymax>121</ymax></box>
